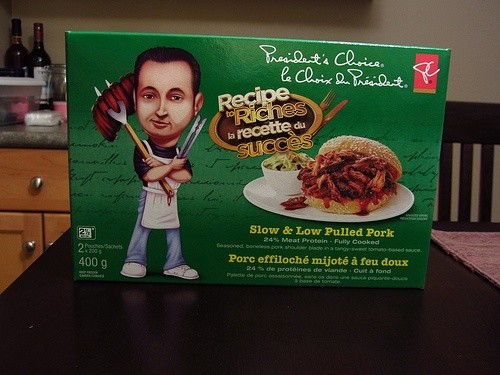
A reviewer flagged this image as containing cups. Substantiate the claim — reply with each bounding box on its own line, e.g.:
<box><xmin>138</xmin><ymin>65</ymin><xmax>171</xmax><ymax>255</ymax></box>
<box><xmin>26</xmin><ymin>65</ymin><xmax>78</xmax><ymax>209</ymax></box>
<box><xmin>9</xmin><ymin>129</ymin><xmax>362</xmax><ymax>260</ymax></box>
<box><xmin>50</xmin><ymin>64</ymin><xmax>67</xmax><ymax>103</ymax></box>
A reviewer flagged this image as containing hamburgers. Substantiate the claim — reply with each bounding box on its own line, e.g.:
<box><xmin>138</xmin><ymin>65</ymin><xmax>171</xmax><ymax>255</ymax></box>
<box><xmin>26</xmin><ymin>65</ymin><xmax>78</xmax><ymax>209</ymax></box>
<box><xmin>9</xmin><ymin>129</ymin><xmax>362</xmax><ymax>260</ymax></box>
<box><xmin>296</xmin><ymin>135</ymin><xmax>402</xmax><ymax>214</ymax></box>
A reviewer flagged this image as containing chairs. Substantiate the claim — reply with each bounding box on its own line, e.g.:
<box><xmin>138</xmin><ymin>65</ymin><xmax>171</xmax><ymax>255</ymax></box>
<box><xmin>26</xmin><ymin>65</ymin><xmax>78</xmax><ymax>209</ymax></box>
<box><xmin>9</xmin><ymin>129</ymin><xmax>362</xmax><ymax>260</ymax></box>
<box><xmin>437</xmin><ymin>101</ymin><xmax>500</xmax><ymax>223</ymax></box>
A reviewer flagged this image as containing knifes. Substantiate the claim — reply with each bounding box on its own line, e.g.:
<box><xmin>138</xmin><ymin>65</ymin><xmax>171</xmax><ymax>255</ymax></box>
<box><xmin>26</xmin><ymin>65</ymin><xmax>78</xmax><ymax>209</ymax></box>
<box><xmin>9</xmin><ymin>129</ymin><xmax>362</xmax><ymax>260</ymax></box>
<box><xmin>311</xmin><ymin>99</ymin><xmax>348</xmax><ymax>136</ymax></box>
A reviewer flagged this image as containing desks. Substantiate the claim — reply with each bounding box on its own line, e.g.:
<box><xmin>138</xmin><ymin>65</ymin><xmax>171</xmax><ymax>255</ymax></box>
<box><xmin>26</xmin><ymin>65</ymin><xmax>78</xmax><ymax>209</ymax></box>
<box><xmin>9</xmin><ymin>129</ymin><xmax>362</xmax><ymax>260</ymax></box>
<box><xmin>0</xmin><ymin>221</ymin><xmax>500</xmax><ymax>375</ymax></box>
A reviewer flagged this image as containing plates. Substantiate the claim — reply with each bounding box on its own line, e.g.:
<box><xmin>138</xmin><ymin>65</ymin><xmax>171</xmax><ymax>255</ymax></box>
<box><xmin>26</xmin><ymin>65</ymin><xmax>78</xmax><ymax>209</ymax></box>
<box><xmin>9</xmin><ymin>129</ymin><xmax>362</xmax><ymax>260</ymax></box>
<box><xmin>243</xmin><ymin>176</ymin><xmax>415</xmax><ymax>223</ymax></box>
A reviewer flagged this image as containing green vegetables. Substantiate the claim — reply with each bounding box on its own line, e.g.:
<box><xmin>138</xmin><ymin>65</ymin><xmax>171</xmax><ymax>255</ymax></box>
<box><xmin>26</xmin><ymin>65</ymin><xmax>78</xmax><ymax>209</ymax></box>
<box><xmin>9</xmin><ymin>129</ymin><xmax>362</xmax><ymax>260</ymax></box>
<box><xmin>263</xmin><ymin>152</ymin><xmax>312</xmax><ymax>170</ymax></box>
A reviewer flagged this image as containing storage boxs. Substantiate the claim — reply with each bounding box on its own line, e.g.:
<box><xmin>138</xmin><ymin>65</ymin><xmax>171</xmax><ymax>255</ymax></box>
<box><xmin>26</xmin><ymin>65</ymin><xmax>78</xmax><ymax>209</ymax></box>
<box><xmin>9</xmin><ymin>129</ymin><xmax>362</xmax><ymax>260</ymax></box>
<box><xmin>0</xmin><ymin>77</ymin><xmax>47</xmax><ymax>125</ymax></box>
<box><xmin>64</xmin><ymin>31</ymin><xmax>450</xmax><ymax>289</ymax></box>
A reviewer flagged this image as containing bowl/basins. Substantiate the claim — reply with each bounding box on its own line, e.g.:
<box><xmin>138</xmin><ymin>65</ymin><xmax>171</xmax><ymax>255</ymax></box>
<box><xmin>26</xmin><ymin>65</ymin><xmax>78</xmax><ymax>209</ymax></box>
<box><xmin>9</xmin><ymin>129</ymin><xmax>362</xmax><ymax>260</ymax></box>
<box><xmin>262</xmin><ymin>158</ymin><xmax>313</xmax><ymax>195</ymax></box>
<box><xmin>0</xmin><ymin>76</ymin><xmax>46</xmax><ymax>126</ymax></box>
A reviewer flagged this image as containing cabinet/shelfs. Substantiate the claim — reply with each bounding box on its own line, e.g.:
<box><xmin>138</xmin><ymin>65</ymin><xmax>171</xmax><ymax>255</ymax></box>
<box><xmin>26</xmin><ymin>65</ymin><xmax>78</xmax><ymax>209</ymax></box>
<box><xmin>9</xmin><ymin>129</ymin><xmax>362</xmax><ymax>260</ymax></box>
<box><xmin>0</xmin><ymin>148</ymin><xmax>71</xmax><ymax>294</ymax></box>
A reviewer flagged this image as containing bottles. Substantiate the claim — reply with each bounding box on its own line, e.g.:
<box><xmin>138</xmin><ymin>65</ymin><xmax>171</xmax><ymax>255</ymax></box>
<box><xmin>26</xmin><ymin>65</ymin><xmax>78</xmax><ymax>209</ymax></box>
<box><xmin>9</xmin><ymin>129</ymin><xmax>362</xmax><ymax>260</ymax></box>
<box><xmin>26</xmin><ymin>22</ymin><xmax>53</xmax><ymax>111</ymax></box>
<box><xmin>4</xmin><ymin>19</ymin><xmax>29</xmax><ymax>76</ymax></box>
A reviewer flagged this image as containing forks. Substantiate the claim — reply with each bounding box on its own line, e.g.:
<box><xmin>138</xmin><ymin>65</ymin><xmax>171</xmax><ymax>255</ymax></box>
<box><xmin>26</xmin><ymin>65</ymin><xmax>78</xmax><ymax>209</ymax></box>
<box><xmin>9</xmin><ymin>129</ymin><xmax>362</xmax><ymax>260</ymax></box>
<box><xmin>319</xmin><ymin>89</ymin><xmax>337</xmax><ymax>111</ymax></box>
